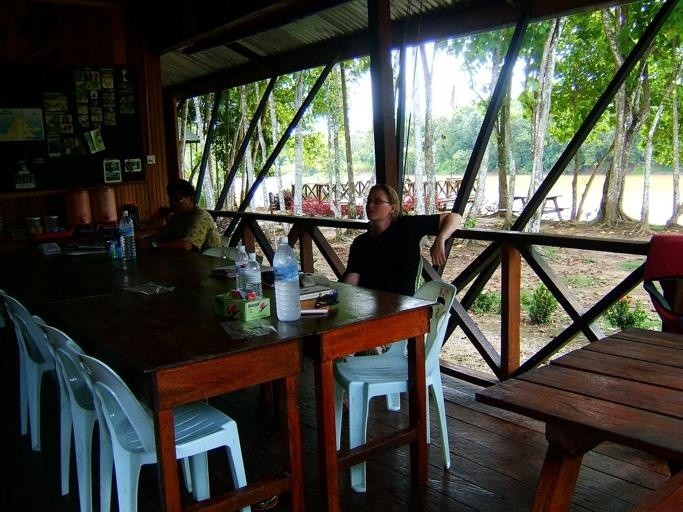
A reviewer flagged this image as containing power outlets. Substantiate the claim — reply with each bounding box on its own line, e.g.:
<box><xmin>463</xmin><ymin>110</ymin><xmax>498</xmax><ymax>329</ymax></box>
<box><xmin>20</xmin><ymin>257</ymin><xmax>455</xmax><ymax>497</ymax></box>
<box><xmin>147</xmin><ymin>155</ymin><xmax>156</xmax><ymax>165</ymax></box>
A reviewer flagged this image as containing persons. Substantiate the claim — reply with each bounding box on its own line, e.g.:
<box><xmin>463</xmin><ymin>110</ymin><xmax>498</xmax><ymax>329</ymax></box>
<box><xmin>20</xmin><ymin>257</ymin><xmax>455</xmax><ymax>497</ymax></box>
<box><xmin>342</xmin><ymin>183</ymin><xmax>461</xmax><ymax>297</ymax></box>
<box><xmin>133</xmin><ymin>178</ymin><xmax>220</xmax><ymax>255</ymax></box>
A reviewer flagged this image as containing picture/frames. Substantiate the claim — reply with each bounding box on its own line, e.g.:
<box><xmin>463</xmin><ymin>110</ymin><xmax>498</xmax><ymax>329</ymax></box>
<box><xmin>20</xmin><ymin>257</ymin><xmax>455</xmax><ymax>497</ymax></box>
<box><xmin>125</xmin><ymin>159</ymin><xmax>142</xmax><ymax>172</ymax></box>
<box><xmin>103</xmin><ymin>159</ymin><xmax>123</xmax><ymax>183</ymax></box>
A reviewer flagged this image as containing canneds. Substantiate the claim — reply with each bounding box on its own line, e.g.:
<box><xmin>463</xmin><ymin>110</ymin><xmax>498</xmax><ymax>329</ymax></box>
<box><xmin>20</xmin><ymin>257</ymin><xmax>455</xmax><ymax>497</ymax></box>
<box><xmin>26</xmin><ymin>217</ymin><xmax>41</xmax><ymax>231</ymax></box>
<box><xmin>106</xmin><ymin>241</ymin><xmax>117</xmax><ymax>258</ymax></box>
<box><xmin>44</xmin><ymin>216</ymin><xmax>58</xmax><ymax>230</ymax></box>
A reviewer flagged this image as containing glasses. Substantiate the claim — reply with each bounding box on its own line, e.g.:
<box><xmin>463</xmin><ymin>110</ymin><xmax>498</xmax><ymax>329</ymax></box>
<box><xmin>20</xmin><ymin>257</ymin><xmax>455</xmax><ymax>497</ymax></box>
<box><xmin>367</xmin><ymin>199</ymin><xmax>392</xmax><ymax>205</ymax></box>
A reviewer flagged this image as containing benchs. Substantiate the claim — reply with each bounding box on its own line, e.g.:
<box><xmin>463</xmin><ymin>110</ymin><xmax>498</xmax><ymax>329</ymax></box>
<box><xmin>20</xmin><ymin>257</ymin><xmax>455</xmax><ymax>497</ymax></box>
<box><xmin>496</xmin><ymin>208</ymin><xmax>526</xmax><ymax>212</ymax></box>
<box><xmin>522</xmin><ymin>205</ymin><xmax>569</xmax><ymax>210</ymax></box>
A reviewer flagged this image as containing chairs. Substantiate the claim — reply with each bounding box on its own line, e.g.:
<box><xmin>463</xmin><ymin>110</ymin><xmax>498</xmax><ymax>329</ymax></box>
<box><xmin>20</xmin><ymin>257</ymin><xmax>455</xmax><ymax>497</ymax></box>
<box><xmin>1</xmin><ymin>226</ymin><xmax>435</xmax><ymax>511</ymax></box>
<box><xmin>334</xmin><ymin>279</ymin><xmax>457</xmax><ymax>494</ymax></box>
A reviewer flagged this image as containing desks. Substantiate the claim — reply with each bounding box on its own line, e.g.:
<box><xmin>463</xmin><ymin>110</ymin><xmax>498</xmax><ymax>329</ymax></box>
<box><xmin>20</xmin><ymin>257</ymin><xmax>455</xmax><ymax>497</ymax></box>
<box><xmin>1</xmin><ymin>225</ymin><xmax>435</xmax><ymax>511</ymax></box>
<box><xmin>474</xmin><ymin>327</ymin><xmax>683</xmax><ymax>512</ymax></box>
<box><xmin>504</xmin><ymin>195</ymin><xmax>563</xmax><ymax>225</ymax></box>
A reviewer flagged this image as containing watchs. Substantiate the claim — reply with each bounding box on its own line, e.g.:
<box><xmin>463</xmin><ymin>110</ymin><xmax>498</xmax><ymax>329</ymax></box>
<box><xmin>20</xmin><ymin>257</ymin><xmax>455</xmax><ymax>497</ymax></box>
<box><xmin>150</xmin><ymin>240</ymin><xmax>158</xmax><ymax>250</ymax></box>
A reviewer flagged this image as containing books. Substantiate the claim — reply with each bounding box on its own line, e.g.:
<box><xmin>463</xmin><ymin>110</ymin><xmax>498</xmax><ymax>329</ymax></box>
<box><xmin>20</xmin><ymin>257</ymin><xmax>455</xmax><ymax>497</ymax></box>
<box><xmin>300</xmin><ymin>298</ymin><xmax>330</xmax><ymax>315</ymax></box>
<box><xmin>60</xmin><ymin>245</ymin><xmax>107</xmax><ymax>257</ymax></box>
<box><xmin>299</xmin><ymin>284</ymin><xmax>335</xmax><ymax>302</ymax></box>
<box><xmin>75</xmin><ymin>242</ymin><xmax>104</xmax><ymax>249</ymax></box>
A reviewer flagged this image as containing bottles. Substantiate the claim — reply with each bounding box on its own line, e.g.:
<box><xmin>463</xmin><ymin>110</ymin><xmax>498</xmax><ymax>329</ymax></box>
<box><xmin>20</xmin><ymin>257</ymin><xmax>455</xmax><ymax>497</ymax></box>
<box><xmin>118</xmin><ymin>210</ymin><xmax>136</xmax><ymax>262</ymax></box>
<box><xmin>234</xmin><ymin>244</ymin><xmax>249</xmax><ymax>289</ymax></box>
<box><xmin>27</xmin><ymin>217</ymin><xmax>42</xmax><ymax>234</ymax></box>
<box><xmin>273</xmin><ymin>235</ymin><xmax>301</xmax><ymax>323</ymax></box>
<box><xmin>44</xmin><ymin>215</ymin><xmax>57</xmax><ymax>233</ymax></box>
<box><xmin>244</xmin><ymin>252</ymin><xmax>263</xmax><ymax>296</ymax></box>
